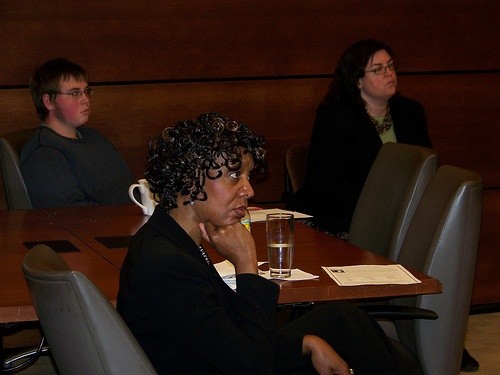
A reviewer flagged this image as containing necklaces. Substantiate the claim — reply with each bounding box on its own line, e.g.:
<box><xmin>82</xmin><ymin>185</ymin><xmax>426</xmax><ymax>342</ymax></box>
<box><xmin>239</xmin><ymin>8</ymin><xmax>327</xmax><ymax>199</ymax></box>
<box><xmin>199</xmin><ymin>246</ymin><xmax>209</xmax><ymax>265</ymax></box>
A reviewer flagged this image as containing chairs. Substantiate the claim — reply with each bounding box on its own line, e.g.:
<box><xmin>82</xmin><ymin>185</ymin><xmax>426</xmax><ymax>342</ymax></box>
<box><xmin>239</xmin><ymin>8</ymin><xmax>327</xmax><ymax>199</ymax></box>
<box><xmin>0</xmin><ymin>128</ymin><xmax>36</xmax><ymax>209</ymax></box>
<box><xmin>285</xmin><ymin>142</ymin><xmax>311</xmax><ymax>196</ymax></box>
<box><xmin>22</xmin><ymin>244</ymin><xmax>158</xmax><ymax>375</ymax></box>
<box><xmin>346</xmin><ymin>142</ymin><xmax>482</xmax><ymax>375</ymax></box>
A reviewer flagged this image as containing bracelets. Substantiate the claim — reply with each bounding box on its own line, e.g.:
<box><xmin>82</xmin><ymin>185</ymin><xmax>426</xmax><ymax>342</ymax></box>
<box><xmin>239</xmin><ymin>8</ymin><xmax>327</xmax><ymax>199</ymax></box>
<box><xmin>369</xmin><ymin>105</ymin><xmax>392</xmax><ymax>134</ymax></box>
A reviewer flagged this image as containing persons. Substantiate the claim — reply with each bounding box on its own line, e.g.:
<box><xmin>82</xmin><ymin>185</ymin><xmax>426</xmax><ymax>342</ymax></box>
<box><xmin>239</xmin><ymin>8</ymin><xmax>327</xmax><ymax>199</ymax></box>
<box><xmin>114</xmin><ymin>115</ymin><xmax>414</xmax><ymax>375</ymax></box>
<box><xmin>21</xmin><ymin>59</ymin><xmax>141</xmax><ymax>208</ymax></box>
<box><xmin>302</xmin><ymin>39</ymin><xmax>480</xmax><ymax>371</ymax></box>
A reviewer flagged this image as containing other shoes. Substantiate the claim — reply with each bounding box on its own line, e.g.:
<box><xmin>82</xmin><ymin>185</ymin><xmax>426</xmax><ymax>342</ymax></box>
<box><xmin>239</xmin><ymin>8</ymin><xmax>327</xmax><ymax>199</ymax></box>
<box><xmin>461</xmin><ymin>348</ymin><xmax>479</xmax><ymax>372</ymax></box>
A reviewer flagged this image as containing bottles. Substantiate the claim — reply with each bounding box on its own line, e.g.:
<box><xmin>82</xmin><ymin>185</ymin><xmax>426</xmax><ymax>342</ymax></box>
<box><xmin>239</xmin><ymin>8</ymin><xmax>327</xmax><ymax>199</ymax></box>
<box><xmin>239</xmin><ymin>209</ymin><xmax>251</xmax><ymax>234</ymax></box>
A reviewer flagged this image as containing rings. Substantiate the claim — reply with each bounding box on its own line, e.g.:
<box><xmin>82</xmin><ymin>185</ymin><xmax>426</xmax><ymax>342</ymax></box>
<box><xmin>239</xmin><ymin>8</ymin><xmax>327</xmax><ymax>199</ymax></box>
<box><xmin>349</xmin><ymin>369</ymin><xmax>354</xmax><ymax>374</ymax></box>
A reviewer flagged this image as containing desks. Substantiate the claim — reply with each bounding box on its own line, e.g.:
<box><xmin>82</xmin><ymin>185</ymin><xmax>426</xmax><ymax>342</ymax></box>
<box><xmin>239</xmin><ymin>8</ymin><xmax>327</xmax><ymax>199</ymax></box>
<box><xmin>0</xmin><ymin>208</ymin><xmax>120</xmax><ymax>375</ymax></box>
<box><xmin>46</xmin><ymin>206</ymin><xmax>442</xmax><ymax>312</ymax></box>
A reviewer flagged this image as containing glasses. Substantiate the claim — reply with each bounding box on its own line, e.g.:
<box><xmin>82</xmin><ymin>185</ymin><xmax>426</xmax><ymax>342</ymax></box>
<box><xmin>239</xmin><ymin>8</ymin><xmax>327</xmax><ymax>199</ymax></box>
<box><xmin>367</xmin><ymin>63</ymin><xmax>396</xmax><ymax>75</ymax></box>
<box><xmin>50</xmin><ymin>87</ymin><xmax>94</xmax><ymax>97</ymax></box>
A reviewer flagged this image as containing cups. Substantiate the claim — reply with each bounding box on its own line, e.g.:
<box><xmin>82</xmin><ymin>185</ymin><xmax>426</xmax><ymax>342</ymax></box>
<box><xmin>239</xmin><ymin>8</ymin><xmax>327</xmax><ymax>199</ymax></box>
<box><xmin>266</xmin><ymin>212</ymin><xmax>295</xmax><ymax>278</ymax></box>
<box><xmin>129</xmin><ymin>177</ymin><xmax>163</xmax><ymax>214</ymax></box>
